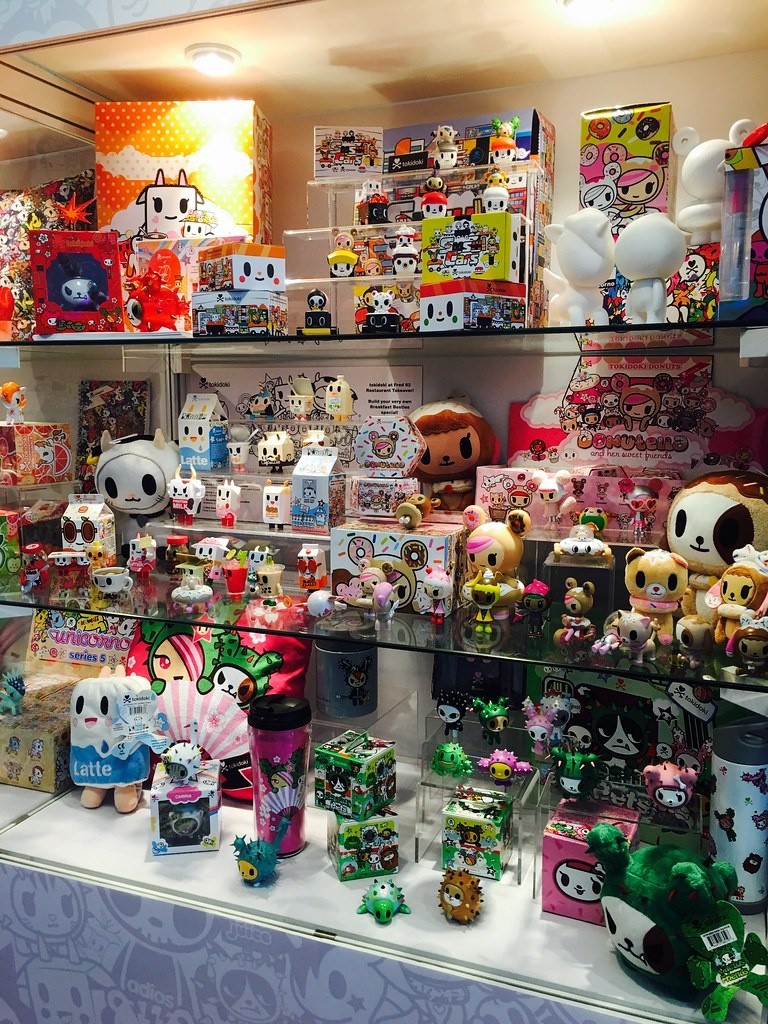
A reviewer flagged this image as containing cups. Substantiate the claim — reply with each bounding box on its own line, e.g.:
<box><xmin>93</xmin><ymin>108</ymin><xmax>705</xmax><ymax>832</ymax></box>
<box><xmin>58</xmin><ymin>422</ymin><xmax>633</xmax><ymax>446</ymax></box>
<box><xmin>91</xmin><ymin>567</ymin><xmax>133</xmax><ymax>593</ymax></box>
<box><xmin>707</xmin><ymin>717</ymin><xmax>768</xmax><ymax>916</ymax></box>
<box><xmin>222</xmin><ymin>560</ymin><xmax>250</xmax><ymax>593</ymax></box>
<box><xmin>226</xmin><ymin>442</ymin><xmax>251</xmax><ymax>475</ymax></box>
<box><xmin>254</xmin><ymin>563</ymin><xmax>285</xmax><ymax>598</ymax></box>
<box><xmin>313</xmin><ymin>639</ymin><xmax>379</xmax><ymax>718</ymax></box>
<box><xmin>287</xmin><ymin>395</ymin><xmax>314</xmax><ymax>421</ymax></box>
<box><xmin>247</xmin><ymin>695</ymin><xmax>313</xmax><ymax>855</ymax></box>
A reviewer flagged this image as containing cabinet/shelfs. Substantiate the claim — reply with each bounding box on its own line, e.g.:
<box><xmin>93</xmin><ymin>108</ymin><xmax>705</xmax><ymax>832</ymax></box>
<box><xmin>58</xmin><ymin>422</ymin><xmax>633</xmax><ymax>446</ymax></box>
<box><xmin>0</xmin><ymin>0</ymin><xmax>768</xmax><ymax>1024</ymax></box>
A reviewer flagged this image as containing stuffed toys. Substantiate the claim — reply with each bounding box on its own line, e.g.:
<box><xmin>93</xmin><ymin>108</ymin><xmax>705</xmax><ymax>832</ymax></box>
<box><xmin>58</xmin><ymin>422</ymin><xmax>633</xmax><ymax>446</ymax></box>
<box><xmin>665</xmin><ymin>470</ymin><xmax>768</xmax><ymax>648</ymax></box>
<box><xmin>586</xmin><ymin>822</ymin><xmax>767</xmax><ymax>1021</ymax></box>
<box><xmin>70</xmin><ymin>665</ymin><xmax>151</xmax><ymax>814</ymax></box>
<box><xmin>94</xmin><ymin>429</ymin><xmax>179</xmax><ymax>564</ymax></box>
<box><xmin>405</xmin><ymin>397</ymin><xmax>500</xmax><ymax>513</ymax></box>
<box><xmin>532</xmin><ymin>470</ymin><xmax>577</xmax><ymax>531</ymax></box>
<box><xmin>713</xmin><ymin>566</ymin><xmax>768</xmax><ymax>644</ymax></box>
<box><xmin>624</xmin><ymin>547</ymin><xmax>689</xmax><ymax>646</ymax></box>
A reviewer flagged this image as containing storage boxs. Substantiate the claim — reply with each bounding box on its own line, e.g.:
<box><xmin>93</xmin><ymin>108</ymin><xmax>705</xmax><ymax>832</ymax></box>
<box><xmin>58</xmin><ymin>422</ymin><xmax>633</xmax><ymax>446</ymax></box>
<box><xmin>0</xmin><ymin>98</ymin><xmax>768</xmax><ymax>930</ymax></box>
